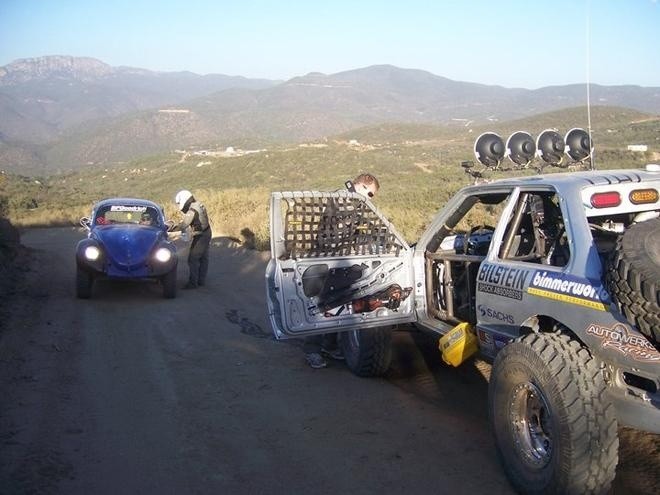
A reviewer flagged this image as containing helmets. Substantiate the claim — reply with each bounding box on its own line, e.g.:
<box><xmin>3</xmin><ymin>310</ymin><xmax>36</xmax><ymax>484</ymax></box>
<box><xmin>175</xmin><ymin>190</ymin><xmax>193</xmax><ymax>211</ymax></box>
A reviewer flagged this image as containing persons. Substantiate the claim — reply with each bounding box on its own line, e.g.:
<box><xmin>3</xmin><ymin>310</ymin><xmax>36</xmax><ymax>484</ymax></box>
<box><xmin>164</xmin><ymin>190</ymin><xmax>212</xmax><ymax>289</ymax></box>
<box><xmin>141</xmin><ymin>213</ymin><xmax>153</xmax><ymax>226</ymax></box>
<box><xmin>301</xmin><ymin>174</ymin><xmax>379</xmax><ymax>368</ymax></box>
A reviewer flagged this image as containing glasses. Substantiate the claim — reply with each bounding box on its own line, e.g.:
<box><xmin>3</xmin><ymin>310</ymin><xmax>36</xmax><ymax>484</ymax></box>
<box><xmin>362</xmin><ymin>185</ymin><xmax>374</xmax><ymax>198</ymax></box>
<box><xmin>142</xmin><ymin>218</ymin><xmax>150</xmax><ymax>222</ymax></box>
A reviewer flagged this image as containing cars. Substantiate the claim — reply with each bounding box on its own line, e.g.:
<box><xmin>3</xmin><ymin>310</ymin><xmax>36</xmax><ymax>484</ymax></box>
<box><xmin>75</xmin><ymin>198</ymin><xmax>179</xmax><ymax>299</ymax></box>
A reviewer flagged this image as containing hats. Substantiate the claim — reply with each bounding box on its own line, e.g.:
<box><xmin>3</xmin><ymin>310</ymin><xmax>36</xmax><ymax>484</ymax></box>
<box><xmin>140</xmin><ymin>212</ymin><xmax>154</xmax><ymax>219</ymax></box>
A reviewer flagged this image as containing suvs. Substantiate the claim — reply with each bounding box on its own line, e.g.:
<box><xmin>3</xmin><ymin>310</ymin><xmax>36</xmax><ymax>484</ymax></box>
<box><xmin>265</xmin><ymin>128</ymin><xmax>660</xmax><ymax>494</ymax></box>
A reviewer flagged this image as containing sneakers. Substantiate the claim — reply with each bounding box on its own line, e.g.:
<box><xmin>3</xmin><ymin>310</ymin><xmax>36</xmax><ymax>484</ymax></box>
<box><xmin>180</xmin><ymin>283</ymin><xmax>205</xmax><ymax>290</ymax></box>
<box><xmin>320</xmin><ymin>346</ymin><xmax>345</xmax><ymax>360</ymax></box>
<box><xmin>303</xmin><ymin>352</ymin><xmax>328</xmax><ymax>369</ymax></box>
